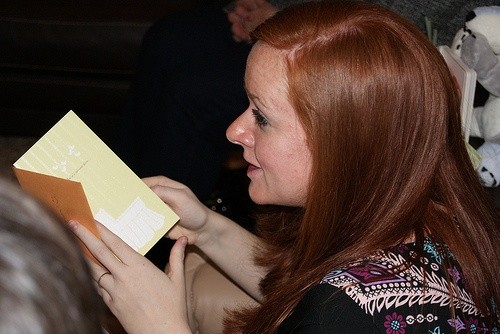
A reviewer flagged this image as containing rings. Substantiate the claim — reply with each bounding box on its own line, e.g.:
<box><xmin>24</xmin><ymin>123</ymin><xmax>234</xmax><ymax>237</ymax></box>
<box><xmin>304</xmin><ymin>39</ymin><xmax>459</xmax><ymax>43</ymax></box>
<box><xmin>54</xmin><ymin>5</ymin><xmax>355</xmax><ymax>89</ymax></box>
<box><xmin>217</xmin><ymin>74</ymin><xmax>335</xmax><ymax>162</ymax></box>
<box><xmin>97</xmin><ymin>272</ymin><xmax>112</xmax><ymax>288</ymax></box>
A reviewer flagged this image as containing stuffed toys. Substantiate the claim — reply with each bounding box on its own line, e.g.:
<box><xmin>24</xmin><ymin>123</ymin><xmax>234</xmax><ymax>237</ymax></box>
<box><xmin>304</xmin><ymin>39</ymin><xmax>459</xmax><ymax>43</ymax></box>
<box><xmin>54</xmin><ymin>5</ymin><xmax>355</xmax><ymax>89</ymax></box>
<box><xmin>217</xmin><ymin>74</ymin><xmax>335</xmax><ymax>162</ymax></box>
<box><xmin>452</xmin><ymin>6</ymin><xmax>500</xmax><ymax>188</ymax></box>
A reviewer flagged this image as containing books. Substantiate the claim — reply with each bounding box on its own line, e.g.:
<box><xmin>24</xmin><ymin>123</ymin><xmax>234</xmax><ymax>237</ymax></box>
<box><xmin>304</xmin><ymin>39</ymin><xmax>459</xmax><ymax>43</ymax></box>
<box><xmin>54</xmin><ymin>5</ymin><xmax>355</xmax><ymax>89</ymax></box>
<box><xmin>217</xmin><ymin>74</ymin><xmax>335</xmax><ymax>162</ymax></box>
<box><xmin>437</xmin><ymin>45</ymin><xmax>477</xmax><ymax>143</ymax></box>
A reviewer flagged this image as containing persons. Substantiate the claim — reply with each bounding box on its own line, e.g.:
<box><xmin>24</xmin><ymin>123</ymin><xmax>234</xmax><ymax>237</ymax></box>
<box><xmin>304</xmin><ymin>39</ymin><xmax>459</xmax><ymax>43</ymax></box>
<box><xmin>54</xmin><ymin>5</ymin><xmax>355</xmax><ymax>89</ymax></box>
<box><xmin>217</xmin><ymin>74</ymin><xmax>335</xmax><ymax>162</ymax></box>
<box><xmin>229</xmin><ymin>0</ymin><xmax>500</xmax><ymax>48</ymax></box>
<box><xmin>68</xmin><ymin>0</ymin><xmax>500</xmax><ymax>334</ymax></box>
